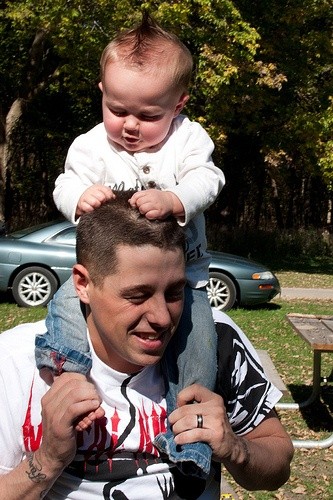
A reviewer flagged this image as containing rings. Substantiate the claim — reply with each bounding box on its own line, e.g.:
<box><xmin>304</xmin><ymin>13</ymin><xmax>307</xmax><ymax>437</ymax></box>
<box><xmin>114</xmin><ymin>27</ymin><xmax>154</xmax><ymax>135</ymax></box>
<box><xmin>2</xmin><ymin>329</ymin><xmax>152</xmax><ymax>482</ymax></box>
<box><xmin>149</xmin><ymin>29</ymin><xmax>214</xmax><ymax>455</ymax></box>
<box><xmin>197</xmin><ymin>414</ymin><xmax>203</xmax><ymax>428</ymax></box>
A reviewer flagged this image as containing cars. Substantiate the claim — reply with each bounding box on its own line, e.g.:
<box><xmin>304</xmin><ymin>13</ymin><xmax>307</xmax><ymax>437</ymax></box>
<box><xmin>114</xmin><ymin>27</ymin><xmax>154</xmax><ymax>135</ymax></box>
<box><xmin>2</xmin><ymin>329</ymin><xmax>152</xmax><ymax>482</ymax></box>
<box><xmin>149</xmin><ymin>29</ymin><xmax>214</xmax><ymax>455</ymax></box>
<box><xmin>0</xmin><ymin>218</ymin><xmax>281</xmax><ymax>312</ymax></box>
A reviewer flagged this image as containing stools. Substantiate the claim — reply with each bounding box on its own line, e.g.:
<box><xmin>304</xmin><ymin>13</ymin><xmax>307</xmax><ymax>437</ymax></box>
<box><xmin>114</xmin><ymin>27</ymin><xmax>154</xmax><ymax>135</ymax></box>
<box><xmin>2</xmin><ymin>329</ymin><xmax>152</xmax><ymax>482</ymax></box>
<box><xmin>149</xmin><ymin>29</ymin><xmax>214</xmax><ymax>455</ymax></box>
<box><xmin>284</xmin><ymin>313</ymin><xmax>333</xmax><ymax>387</ymax></box>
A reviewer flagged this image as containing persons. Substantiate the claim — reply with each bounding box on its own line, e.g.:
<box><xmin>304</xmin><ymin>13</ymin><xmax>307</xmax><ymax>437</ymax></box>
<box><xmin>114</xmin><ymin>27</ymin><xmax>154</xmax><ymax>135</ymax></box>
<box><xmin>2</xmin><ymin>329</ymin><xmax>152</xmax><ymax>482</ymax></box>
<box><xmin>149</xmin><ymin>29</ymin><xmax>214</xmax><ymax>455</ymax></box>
<box><xmin>0</xmin><ymin>190</ymin><xmax>295</xmax><ymax>500</ymax></box>
<box><xmin>33</xmin><ymin>22</ymin><xmax>226</xmax><ymax>479</ymax></box>
